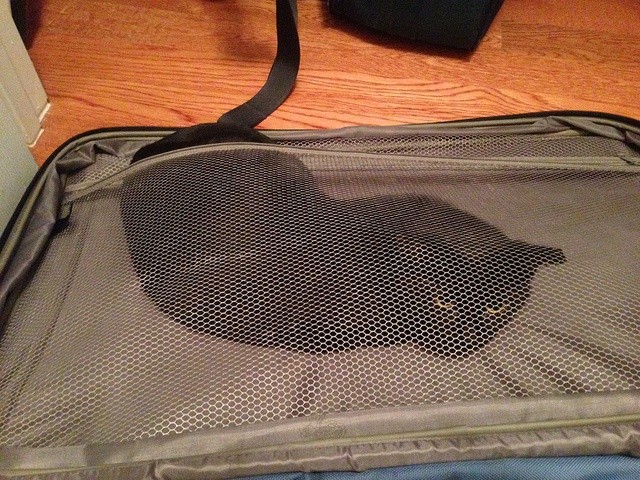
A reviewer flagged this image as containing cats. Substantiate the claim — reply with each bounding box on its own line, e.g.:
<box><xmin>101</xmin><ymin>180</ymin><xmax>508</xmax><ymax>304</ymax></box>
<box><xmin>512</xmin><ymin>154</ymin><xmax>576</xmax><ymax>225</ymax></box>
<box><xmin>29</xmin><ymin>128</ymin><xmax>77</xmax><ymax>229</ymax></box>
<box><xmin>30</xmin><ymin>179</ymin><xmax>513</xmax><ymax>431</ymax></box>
<box><xmin>122</xmin><ymin>123</ymin><xmax>569</xmax><ymax>359</ymax></box>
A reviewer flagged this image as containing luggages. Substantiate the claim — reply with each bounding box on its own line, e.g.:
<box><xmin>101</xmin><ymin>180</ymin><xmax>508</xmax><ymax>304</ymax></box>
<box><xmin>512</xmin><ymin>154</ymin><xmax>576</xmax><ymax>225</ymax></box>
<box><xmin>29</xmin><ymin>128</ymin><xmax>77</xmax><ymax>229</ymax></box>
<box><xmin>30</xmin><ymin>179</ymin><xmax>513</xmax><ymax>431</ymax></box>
<box><xmin>2</xmin><ymin>107</ymin><xmax>640</xmax><ymax>478</ymax></box>
